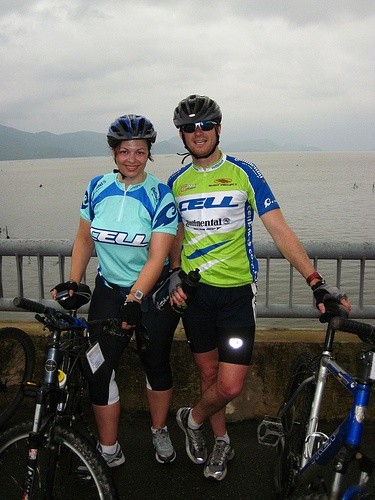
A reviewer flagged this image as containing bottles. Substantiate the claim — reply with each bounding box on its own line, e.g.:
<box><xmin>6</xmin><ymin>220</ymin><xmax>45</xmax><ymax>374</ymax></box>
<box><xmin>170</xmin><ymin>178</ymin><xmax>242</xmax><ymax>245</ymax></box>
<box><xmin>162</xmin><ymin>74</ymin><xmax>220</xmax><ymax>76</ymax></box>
<box><xmin>170</xmin><ymin>267</ymin><xmax>202</xmax><ymax>315</ymax></box>
<box><xmin>57</xmin><ymin>369</ymin><xmax>69</xmax><ymax>411</ymax></box>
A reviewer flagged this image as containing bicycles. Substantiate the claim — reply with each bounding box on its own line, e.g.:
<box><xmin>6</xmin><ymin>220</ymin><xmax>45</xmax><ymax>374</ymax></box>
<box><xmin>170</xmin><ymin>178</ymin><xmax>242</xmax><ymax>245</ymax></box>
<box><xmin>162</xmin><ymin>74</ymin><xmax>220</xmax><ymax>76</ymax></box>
<box><xmin>256</xmin><ymin>298</ymin><xmax>375</xmax><ymax>500</ymax></box>
<box><xmin>0</xmin><ymin>301</ymin><xmax>136</xmax><ymax>500</ymax></box>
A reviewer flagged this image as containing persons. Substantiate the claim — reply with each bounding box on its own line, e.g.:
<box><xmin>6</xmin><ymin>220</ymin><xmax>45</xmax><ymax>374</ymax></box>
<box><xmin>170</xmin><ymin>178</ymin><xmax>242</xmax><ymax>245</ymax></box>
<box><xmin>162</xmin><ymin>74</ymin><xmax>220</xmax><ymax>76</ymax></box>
<box><xmin>167</xmin><ymin>92</ymin><xmax>351</xmax><ymax>481</ymax></box>
<box><xmin>50</xmin><ymin>114</ymin><xmax>178</xmax><ymax>480</ymax></box>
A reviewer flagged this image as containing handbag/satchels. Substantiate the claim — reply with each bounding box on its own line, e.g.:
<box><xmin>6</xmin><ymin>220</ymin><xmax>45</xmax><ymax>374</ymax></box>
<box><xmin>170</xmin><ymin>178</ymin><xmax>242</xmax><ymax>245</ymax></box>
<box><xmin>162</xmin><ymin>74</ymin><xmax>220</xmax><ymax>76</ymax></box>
<box><xmin>147</xmin><ymin>281</ymin><xmax>173</xmax><ymax>314</ymax></box>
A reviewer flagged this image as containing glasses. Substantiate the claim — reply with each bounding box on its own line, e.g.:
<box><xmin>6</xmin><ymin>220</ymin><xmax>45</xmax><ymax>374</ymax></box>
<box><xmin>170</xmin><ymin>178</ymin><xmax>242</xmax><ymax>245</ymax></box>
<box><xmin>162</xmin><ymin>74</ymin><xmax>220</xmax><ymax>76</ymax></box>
<box><xmin>181</xmin><ymin>121</ymin><xmax>217</xmax><ymax>133</ymax></box>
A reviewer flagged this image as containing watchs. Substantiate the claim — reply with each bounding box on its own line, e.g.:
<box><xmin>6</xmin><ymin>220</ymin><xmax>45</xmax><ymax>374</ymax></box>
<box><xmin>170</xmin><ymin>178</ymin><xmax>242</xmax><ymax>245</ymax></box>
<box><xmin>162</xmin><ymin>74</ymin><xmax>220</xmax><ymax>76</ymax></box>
<box><xmin>306</xmin><ymin>271</ymin><xmax>324</xmax><ymax>286</ymax></box>
<box><xmin>130</xmin><ymin>289</ymin><xmax>145</xmax><ymax>302</ymax></box>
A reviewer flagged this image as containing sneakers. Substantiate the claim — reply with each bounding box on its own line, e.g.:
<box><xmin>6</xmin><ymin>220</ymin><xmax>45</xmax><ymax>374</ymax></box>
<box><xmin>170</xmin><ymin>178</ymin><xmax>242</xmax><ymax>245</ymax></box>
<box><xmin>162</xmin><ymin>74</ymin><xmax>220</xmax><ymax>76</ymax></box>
<box><xmin>150</xmin><ymin>424</ymin><xmax>176</xmax><ymax>464</ymax></box>
<box><xmin>176</xmin><ymin>407</ymin><xmax>208</xmax><ymax>465</ymax></box>
<box><xmin>71</xmin><ymin>439</ymin><xmax>125</xmax><ymax>471</ymax></box>
<box><xmin>203</xmin><ymin>438</ymin><xmax>235</xmax><ymax>481</ymax></box>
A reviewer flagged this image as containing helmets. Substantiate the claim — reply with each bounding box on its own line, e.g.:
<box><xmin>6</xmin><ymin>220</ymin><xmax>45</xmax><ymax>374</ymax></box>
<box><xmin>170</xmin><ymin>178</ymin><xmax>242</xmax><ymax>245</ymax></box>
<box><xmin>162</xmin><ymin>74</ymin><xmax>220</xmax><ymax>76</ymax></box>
<box><xmin>107</xmin><ymin>115</ymin><xmax>157</xmax><ymax>147</ymax></box>
<box><xmin>173</xmin><ymin>95</ymin><xmax>222</xmax><ymax>129</ymax></box>
